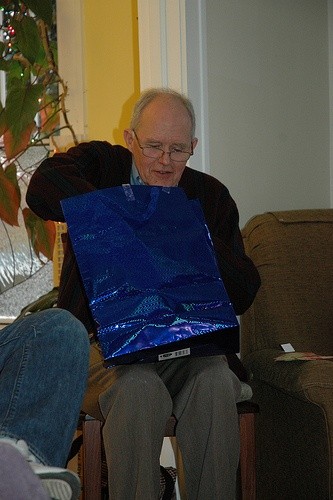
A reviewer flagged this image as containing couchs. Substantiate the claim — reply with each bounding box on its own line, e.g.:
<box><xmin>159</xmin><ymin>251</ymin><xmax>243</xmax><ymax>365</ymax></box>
<box><xmin>240</xmin><ymin>208</ymin><xmax>333</xmax><ymax>500</ymax></box>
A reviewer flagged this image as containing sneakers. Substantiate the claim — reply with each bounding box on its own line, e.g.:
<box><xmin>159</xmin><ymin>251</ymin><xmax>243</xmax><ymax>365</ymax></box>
<box><xmin>0</xmin><ymin>437</ymin><xmax>83</xmax><ymax>500</ymax></box>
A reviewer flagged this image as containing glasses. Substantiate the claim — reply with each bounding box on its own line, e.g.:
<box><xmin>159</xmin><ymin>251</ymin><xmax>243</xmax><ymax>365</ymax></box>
<box><xmin>129</xmin><ymin>126</ymin><xmax>194</xmax><ymax>164</ymax></box>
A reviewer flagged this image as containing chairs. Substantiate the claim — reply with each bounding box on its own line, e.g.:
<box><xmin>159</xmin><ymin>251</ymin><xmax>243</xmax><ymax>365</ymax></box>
<box><xmin>82</xmin><ymin>403</ymin><xmax>255</xmax><ymax>500</ymax></box>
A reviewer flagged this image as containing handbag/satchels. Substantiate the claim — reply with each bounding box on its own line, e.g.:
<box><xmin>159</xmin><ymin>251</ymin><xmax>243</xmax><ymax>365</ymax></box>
<box><xmin>59</xmin><ymin>185</ymin><xmax>241</xmax><ymax>369</ymax></box>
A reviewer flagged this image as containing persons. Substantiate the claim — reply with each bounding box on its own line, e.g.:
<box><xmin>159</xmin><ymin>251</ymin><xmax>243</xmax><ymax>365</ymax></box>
<box><xmin>0</xmin><ymin>308</ymin><xmax>90</xmax><ymax>500</ymax></box>
<box><xmin>25</xmin><ymin>89</ymin><xmax>261</xmax><ymax>500</ymax></box>
